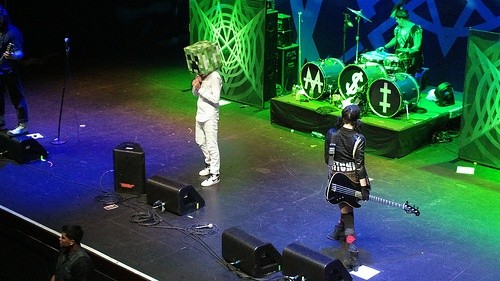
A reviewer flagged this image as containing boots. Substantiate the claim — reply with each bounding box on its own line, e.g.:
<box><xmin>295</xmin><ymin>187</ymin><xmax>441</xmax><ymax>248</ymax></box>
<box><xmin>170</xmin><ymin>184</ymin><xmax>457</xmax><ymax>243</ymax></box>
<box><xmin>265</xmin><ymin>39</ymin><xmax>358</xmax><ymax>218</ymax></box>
<box><xmin>343</xmin><ymin>250</ymin><xmax>359</xmax><ymax>271</ymax></box>
<box><xmin>327</xmin><ymin>220</ymin><xmax>343</xmax><ymax>240</ymax></box>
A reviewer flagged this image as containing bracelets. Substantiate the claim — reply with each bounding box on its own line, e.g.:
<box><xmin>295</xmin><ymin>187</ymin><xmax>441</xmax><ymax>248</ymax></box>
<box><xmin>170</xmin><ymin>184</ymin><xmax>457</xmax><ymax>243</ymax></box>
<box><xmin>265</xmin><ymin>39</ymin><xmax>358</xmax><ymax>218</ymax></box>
<box><xmin>383</xmin><ymin>45</ymin><xmax>386</xmax><ymax>49</ymax></box>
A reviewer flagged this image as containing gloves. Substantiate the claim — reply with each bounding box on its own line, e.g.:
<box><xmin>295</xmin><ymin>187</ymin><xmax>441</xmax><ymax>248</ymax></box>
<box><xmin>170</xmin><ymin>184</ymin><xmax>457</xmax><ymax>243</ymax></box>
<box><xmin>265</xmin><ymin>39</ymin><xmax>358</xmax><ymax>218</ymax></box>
<box><xmin>361</xmin><ymin>185</ymin><xmax>370</xmax><ymax>201</ymax></box>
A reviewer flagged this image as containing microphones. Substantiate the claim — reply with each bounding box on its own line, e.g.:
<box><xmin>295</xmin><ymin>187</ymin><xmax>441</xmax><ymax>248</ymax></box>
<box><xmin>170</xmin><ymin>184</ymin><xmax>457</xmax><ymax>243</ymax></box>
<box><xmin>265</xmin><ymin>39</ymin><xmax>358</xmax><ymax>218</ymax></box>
<box><xmin>64</xmin><ymin>38</ymin><xmax>70</xmax><ymax>52</ymax></box>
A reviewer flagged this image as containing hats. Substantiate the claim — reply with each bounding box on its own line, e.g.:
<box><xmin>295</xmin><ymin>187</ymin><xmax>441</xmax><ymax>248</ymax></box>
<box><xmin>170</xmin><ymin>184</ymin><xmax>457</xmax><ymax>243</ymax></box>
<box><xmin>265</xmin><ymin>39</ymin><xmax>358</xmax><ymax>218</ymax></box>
<box><xmin>342</xmin><ymin>104</ymin><xmax>362</xmax><ymax>122</ymax></box>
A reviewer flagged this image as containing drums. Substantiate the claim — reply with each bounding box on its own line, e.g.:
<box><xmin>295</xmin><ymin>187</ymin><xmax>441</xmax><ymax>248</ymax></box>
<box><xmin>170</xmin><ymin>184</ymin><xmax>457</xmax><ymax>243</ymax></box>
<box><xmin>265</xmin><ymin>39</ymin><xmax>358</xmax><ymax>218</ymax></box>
<box><xmin>300</xmin><ymin>57</ymin><xmax>345</xmax><ymax>100</ymax></box>
<box><xmin>337</xmin><ymin>62</ymin><xmax>386</xmax><ymax>106</ymax></box>
<box><xmin>366</xmin><ymin>73</ymin><xmax>420</xmax><ymax>119</ymax></box>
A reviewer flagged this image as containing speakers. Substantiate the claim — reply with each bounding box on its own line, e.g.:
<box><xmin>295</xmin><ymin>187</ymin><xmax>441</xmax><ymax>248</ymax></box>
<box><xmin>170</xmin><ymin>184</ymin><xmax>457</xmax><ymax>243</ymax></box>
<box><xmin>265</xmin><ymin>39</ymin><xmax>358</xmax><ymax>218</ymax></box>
<box><xmin>0</xmin><ymin>128</ymin><xmax>49</xmax><ymax>165</ymax></box>
<box><xmin>283</xmin><ymin>244</ymin><xmax>353</xmax><ymax>281</ymax></box>
<box><xmin>113</xmin><ymin>141</ymin><xmax>147</xmax><ymax>196</ymax></box>
<box><xmin>146</xmin><ymin>175</ymin><xmax>205</xmax><ymax>215</ymax></box>
<box><xmin>221</xmin><ymin>227</ymin><xmax>283</xmax><ymax>276</ymax></box>
<box><xmin>261</xmin><ymin>7</ymin><xmax>278</xmax><ymax>102</ymax></box>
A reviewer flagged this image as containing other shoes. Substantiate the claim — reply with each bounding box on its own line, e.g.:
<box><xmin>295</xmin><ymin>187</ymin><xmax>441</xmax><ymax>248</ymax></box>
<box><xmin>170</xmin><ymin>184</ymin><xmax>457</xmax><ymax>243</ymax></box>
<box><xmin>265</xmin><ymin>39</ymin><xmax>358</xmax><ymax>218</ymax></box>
<box><xmin>198</xmin><ymin>163</ymin><xmax>211</xmax><ymax>176</ymax></box>
<box><xmin>7</xmin><ymin>122</ymin><xmax>29</xmax><ymax>136</ymax></box>
<box><xmin>200</xmin><ymin>174</ymin><xmax>220</xmax><ymax>186</ymax></box>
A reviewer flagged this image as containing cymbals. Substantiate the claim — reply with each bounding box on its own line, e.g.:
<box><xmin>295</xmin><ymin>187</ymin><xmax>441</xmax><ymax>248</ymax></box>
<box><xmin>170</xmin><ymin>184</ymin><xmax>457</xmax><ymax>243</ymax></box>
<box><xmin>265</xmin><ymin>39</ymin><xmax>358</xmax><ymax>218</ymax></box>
<box><xmin>346</xmin><ymin>6</ymin><xmax>372</xmax><ymax>23</ymax></box>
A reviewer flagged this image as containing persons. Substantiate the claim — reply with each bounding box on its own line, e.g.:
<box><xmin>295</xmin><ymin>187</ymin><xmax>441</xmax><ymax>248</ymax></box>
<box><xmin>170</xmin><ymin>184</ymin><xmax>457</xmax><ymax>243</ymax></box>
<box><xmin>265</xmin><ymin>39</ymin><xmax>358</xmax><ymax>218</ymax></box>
<box><xmin>50</xmin><ymin>224</ymin><xmax>95</xmax><ymax>281</ymax></box>
<box><xmin>0</xmin><ymin>6</ymin><xmax>30</xmax><ymax>136</ymax></box>
<box><xmin>325</xmin><ymin>105</ymin><xmax>370</xmax><ymax>271</ymax></box>
<box><xmin>184</xmin><ymin>41</ymin><xmax>223</xmax><ymax>186</ymax></box>
<box><xmin>376</xmin><ymin>9</ymin><xmax>422</xmax><ymax>73</ymax></box>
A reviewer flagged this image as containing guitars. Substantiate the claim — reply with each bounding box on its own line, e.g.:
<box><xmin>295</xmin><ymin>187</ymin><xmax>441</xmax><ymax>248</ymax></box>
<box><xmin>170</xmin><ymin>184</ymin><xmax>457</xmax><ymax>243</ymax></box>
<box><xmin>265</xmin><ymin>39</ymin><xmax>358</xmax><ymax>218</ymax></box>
<box><xmin>325</xmin><ymin>171</ymin><xmax>421</xmax><ymax>216</ymax></box>
<box><xmin>0</xmin><ymin>43</ymin><xmax>15</xmax><ymax>66</ymax></box>
